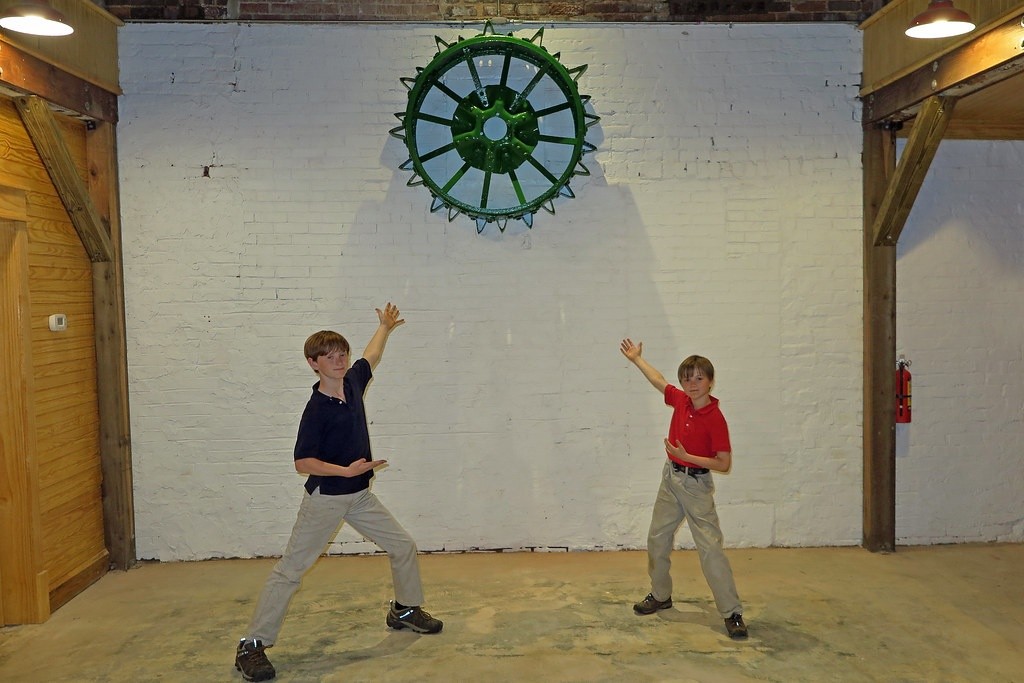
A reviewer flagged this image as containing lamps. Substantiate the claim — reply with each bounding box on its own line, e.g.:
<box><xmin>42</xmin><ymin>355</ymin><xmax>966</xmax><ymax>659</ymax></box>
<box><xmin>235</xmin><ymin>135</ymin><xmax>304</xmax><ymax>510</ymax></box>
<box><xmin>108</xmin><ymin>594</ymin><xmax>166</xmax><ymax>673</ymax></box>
<box><xmin>0</xmin><ymin>0</ymin><xmax>74</xmax><ymax>36</ymax></box>
<box><xmin>905</xmin><ymin>0</ymin><xmax>977</xmax><ymax>39</ymax></box>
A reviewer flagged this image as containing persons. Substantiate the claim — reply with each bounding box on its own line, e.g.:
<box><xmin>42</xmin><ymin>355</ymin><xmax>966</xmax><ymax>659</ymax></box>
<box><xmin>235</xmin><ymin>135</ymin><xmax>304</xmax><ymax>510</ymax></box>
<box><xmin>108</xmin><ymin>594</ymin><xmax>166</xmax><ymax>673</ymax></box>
<box><xmin>234</xmin><ymin>302</ymin><xmax>443</xmax><ymax>683</ymax></box>
<box><xmin>620</xmin><ymin>339</ymin><xmax>748</xmax><ymax>637</ymax></box>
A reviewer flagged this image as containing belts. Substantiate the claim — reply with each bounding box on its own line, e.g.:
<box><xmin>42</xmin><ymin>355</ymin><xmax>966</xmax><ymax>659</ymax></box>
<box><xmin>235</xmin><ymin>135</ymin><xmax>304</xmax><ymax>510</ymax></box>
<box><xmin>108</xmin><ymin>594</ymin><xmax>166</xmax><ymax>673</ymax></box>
<box><xmin>672</xmin><ymin>461</ymin><xmax>710</xmax><ymax>483</ymax></box>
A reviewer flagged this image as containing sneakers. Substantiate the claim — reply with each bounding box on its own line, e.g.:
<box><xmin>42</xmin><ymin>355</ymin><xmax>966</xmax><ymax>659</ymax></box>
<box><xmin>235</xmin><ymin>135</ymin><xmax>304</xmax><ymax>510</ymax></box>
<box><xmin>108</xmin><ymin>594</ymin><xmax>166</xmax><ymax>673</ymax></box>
<box><xmin>724</xmin><ymin>612</ymin><xmax>748</xmax><ymax>639</ymax></box>
<box><xmin>633</xmin><ymin>593</ymin><xmax>672</xmax><ymax>614</ymax></box>
<box><xmin>235</xmin><ymin>638</ymin><xmax>276</xmax><ymax>682</ymax></box>
<box><xmin>386</xmin><ymin>600</ymin><xmax>443</xmax><ymax>634</ymax></box>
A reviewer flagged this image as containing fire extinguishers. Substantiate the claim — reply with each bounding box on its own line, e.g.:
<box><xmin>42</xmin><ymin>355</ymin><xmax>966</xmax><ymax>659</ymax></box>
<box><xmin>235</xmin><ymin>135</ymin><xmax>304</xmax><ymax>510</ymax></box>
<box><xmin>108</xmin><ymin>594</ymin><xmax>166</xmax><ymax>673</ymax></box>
<box><xmin>894</xmin><ymin>354</ymin><xmax>912</xmax><ymax>423</ymax></box>
